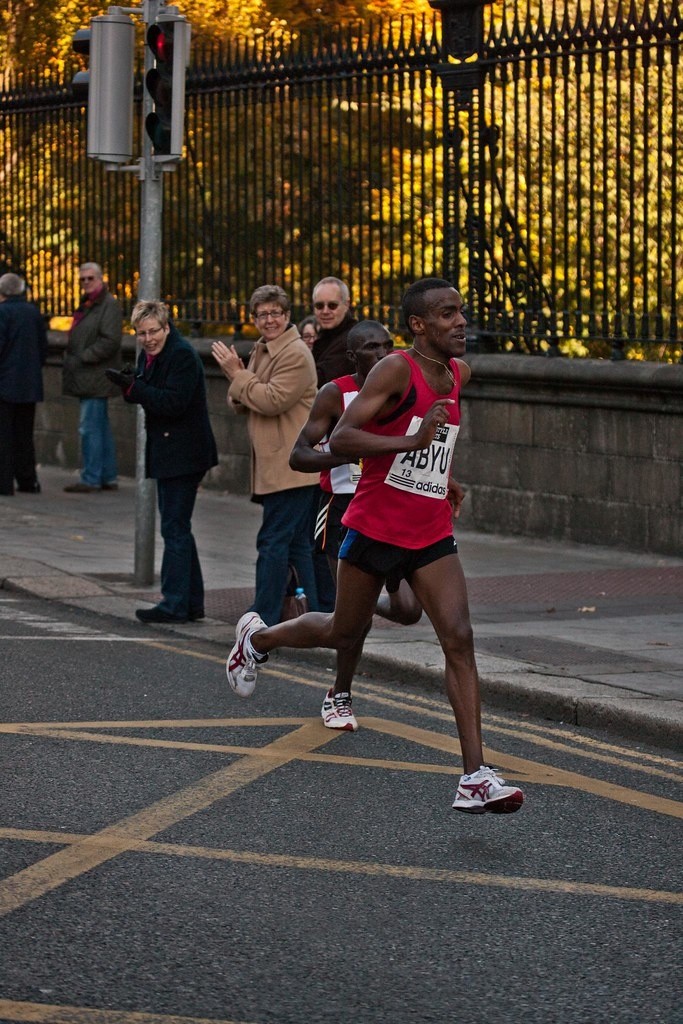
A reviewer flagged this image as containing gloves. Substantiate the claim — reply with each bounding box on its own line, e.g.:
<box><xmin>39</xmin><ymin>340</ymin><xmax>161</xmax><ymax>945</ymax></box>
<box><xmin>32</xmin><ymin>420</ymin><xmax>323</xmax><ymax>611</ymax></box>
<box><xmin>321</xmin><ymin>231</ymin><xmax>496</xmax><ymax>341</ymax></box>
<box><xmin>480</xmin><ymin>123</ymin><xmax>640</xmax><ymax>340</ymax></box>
<box><xmin>104</xmin><ymin>369</ymin><xmax>132</xmax><ymax>388</ymax></box>
<box><xmin>121</xmin><ymin>363</ymin><xmax>141</xmax><ymax>378</ymax></box>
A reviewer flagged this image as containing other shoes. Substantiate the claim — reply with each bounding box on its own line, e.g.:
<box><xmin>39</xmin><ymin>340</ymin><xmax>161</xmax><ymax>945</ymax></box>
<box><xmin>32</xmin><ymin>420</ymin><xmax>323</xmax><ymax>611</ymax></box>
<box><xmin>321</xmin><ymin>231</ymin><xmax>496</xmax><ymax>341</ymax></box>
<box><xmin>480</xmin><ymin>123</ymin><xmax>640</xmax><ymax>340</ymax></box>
<box><xmin>187</xmin><ymin>607</ymin><xmax>205</xmax><ymax>619</ymax></box>
<box><xmin>64</xmin><ymin>483</ymin><xmax>97</xmax><ymax>493</ymax></box>
<box><xmin>17</xmin><ymin>482</ymin><xmax>40</xmax><ymax>493</ymax></box>
<box><xmin>102</xmin><ymin>483</ymin><xmax>119</xmax><ymax>490</ymax></box>
<box><xmin>136</xmin><ymin>606</ymin><xmax>187</xmax><ymax>624</ymax></box>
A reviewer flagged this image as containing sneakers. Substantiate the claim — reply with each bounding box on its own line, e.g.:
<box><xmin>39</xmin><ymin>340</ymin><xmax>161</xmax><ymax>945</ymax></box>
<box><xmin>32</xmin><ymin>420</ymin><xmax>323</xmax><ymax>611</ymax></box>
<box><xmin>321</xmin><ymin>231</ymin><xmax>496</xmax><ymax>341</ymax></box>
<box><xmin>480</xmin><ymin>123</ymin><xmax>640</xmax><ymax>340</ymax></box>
<box><xmin>321</xmin><ymin>689</ymin><xmax>358</xmax><ymax>732</ymax></box>
<box><xmin>226</xmin><ymin>611</ymin><xmax>269</xmax><ymax>697</ymax></box>
<box><xmin>451</xmin><ymin>766</ymin><xmax>524</xmax><ymax>815</ymax></box>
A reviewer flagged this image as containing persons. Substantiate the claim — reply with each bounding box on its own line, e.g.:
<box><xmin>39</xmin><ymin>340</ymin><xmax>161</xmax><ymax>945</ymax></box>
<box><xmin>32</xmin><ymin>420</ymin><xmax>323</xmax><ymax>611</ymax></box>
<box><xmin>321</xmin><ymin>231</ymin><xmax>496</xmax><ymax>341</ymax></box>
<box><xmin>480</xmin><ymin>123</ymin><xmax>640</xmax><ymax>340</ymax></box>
<box><xmin>104</xmin><ymin>300</ymin><xmax>218</xmax><ymax>626</ymax></box>
<box><xmin>211</xmin><ymin>276</ymin><xmax>364</xmax><ymax>629</ymax></box>
<box><xmin>225</xmin><ymin>276</ymin><xmax>522</xmax><ymax>814</ymax></box>
<box><xmin>0</xmin><ymin>273</ymin><xmax>49</xmax><ymax>497</ymax></box>
<box><xmin>60</xmin><ymin>261</ymin><xmax>122</xmax><ymax>494</ymax></box>
<box><xmin>289</xmin><ymin>318</ymin><xmax>423</xmax><ymax>732</ymax></box>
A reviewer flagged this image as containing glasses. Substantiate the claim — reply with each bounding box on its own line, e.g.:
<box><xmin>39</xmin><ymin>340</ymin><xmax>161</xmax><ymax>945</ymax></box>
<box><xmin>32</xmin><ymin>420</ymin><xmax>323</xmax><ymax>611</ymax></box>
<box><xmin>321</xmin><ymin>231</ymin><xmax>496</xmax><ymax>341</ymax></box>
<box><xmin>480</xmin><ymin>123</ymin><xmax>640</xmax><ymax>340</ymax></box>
<box><xmin>314</xmin><ymin>301</ymin><xmax>344</xmax><ymax>310</ymax></box>
<box><xmin>302</xmin><ymin>333</ymin><xmax>318</xmax><ymax>341</ymax></box>
<box><xmin>254</xmin><ymin>311</ymin><xmax>284</xmax><ymax>320</ymax></box>
<box><xmin>80</xmin><ymin>276</ymin><xmax>99</xmax><ymax>282</ymax></box>
<box><xmin>135</xmin><ymin>327</ymin><xmax>163</xmax><ymax>337</ymax></box>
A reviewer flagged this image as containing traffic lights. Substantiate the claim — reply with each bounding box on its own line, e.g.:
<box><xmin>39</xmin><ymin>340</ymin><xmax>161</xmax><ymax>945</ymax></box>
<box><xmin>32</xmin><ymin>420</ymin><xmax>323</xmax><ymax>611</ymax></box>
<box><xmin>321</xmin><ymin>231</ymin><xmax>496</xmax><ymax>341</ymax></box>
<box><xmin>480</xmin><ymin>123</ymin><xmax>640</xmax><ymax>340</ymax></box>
<box><xmin>72</xmin><ymin>17</ymin><xmax>136</xmax><ymax>162</ymax></box>
<box><xmin>145</xmin><ymin>13</ymin><xmax>192</xmax><ymax>172</ymax></box>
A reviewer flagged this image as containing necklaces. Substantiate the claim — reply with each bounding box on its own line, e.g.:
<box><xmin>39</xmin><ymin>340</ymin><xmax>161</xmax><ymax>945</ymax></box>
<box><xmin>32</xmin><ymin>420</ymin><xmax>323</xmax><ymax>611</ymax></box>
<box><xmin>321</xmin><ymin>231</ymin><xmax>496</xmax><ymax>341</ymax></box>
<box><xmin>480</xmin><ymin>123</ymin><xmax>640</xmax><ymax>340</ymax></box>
<box><xmin>412</xmin><ymin>347</ymin><xmax>457</xmax><ymax>385</ymax></box>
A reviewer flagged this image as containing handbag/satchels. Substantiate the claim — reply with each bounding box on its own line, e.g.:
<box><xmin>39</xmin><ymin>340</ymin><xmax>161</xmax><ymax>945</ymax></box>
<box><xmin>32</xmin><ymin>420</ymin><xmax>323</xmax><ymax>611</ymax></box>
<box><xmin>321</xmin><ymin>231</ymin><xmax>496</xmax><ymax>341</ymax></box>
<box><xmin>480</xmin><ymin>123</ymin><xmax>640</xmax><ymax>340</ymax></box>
<box><xmin>282</xmin><ymin>564</ymin><xmax>307</xmax><ymax>622</ymax></box>
